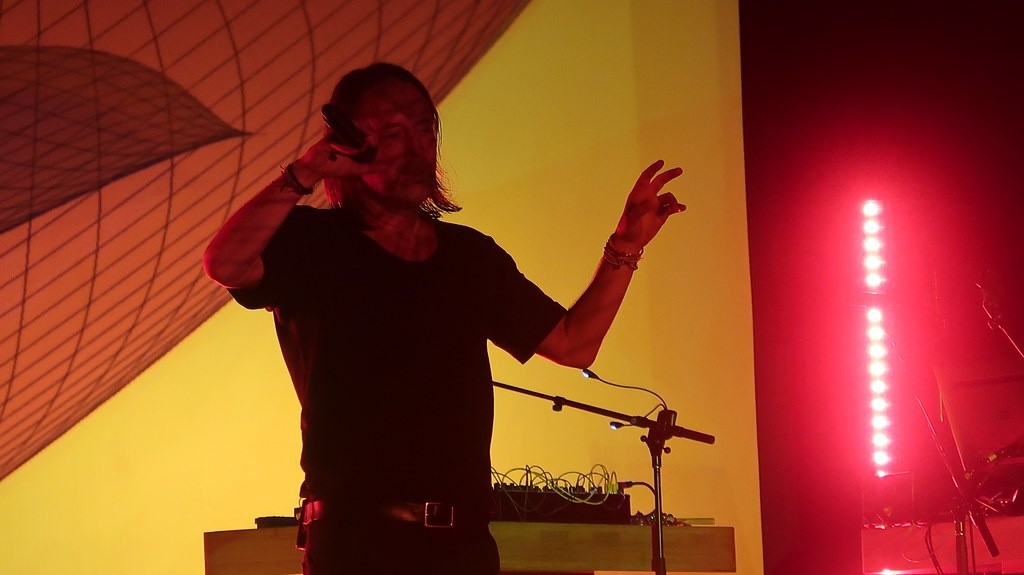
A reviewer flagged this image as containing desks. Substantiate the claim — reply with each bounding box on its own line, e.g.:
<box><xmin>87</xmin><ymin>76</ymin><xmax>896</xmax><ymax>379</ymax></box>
<box><xmin>202</xmin><ymin>522</ymin><xmax>738</xmax><ymax>575</ymax></box>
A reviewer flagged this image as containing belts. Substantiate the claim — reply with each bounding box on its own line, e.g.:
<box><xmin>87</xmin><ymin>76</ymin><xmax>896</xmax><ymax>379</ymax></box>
<box><xmin>304</xmin><ymin>498</ymin><xmax>457</xmax><ymax>529</ymax></box>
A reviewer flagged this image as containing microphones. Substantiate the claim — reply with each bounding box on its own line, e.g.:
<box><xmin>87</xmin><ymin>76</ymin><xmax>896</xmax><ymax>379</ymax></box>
<box><xmin>321</xmin><ymin>104</ymin><xmax>377</xmax><ymax>165</ymax></box>
<box><xmin>845</xmin><ymin>291</ymin><xmax>896</xmax><ymax>308</ymax></box>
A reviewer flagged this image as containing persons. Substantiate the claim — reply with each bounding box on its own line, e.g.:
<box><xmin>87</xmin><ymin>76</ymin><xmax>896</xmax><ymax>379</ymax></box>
<box><xmin>205</xmin><ymin>61</ymin><xmax>686</xmax><ymax>575</ymax></box>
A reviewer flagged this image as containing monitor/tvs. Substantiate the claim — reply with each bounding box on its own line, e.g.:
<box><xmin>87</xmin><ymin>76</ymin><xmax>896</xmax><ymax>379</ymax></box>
<box><xmin>863</xmin><ymin>472</ymin><xmax>915</xmax><ymax>525</ymax></box>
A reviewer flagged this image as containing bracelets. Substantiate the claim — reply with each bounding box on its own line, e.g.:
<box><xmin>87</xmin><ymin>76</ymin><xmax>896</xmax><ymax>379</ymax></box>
<box><xmin>280</xmin><ymin>163</ymin><xmax>314</xmax><ymax>195</ymax></box>
<box><xmin>604</xmin><ymin>234</ymin><xmax>645</xmax><ymax>270</ymax></box>
